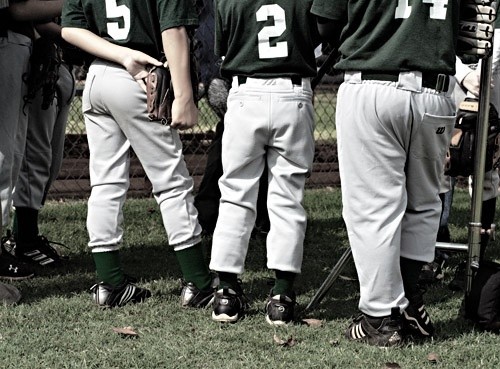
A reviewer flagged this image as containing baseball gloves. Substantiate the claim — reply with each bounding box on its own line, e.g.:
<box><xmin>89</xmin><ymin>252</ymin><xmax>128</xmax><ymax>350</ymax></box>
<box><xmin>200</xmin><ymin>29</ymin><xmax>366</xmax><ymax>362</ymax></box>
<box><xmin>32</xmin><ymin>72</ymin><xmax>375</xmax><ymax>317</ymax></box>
<box><xmin>22</xmin><ymin>36</ymin><xmax>60</xmax><ymax>117</ymax></box>
<box><xmin>149</xmin><ymin>55</ymin><xmax>201</xmax><ymax>128</ymax></box>
<box><xmin>457</xmin><ymin>0</ymin><xmax>497</xmax><ymax>63</ymax></box>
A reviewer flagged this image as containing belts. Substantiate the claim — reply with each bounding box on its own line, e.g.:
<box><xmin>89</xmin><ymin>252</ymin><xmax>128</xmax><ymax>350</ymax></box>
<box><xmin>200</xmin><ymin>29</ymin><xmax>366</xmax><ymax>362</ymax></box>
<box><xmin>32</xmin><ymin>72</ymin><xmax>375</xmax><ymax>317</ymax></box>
<box><xmin>338</xmin><ymin>71</ymin><xmax>450</xmax><ymax>92</ymax></box>
<box><xmin>238</xmin><ymin>74</ymin><xmax>302</xmax><ymax>85</ymax></box>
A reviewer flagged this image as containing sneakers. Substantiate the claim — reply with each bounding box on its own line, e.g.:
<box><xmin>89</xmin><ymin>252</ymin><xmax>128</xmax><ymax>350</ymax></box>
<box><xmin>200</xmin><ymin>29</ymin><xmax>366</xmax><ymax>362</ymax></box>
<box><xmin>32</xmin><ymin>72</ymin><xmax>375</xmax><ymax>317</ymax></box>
<box><xmin>90</xmin><ymin>281</ymin><xmax>151</xmax><ymax>310</ymax></box>
<box><xmin>344</xmin><ymin>307</ymin><xmax>405</xmax><ymax>349</ymax></box>
<box><xmin>180</xmin><ymin>271</ymin><xmax>220</xmax><ymax>309</ymax></box>
<box><xmin>16</xmin><ymin>235</ymin><xmax>71</xmax><ymax>271</ymax></box>
<box><xmin>404</xmin><ymin>283</ymin><xmax>436</xmax><ymax>336</ymax></box>
<box><xmin>414</xmin><ymin>265</ymin><xmax>436</xmax><ymax>282</ymax></box>
<box><xmin>449</xmin><ymin>263</ymin><xmax>466</xmax><ymax>290</ymax></box>
<box><xmin>211</xmin><ymin>278</ymin><xmax>248</xmax><ymax>322</ymax></box>
<box><xmin>266</xmin><ymin>290</ymin><xmax>302</xmax><ymax>325</ymax></box>
<box><xmin>1</xmin><ymin>228</ymin><xmax>35</xmax><ymax>280</ymax></box>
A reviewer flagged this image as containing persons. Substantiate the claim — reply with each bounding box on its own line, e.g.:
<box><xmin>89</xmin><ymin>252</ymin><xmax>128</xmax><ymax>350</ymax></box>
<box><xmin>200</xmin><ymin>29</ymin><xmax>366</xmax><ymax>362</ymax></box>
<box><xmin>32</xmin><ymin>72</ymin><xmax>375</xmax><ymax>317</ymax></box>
<box><xmin>434</xmin><ymin>25</ymin><xmax>500</xmax><ymax>261</ymax></box>
<box><xmin>0</xmin><ymin>0</ymin><xmax>65</xmax><ymax>281</ymax></box>
<box><xmin>209</xmin><ymin>0</ymin><xmax>323</xmax><ymax>324</ymax></box>
<box><xmin>10</xmin><ymin>49</ymin><xmax>76</xmax><ymax>272</ymax></box>
<box><xmin>61</xmin><ymin>0</ymin><xmax>215</xmax><ymax>310</ymax></box>
<box><xmin>310</xmin><ymin>0</ymin><xmax>479</xmax><ymax>350</ymax></box>
<box><xmin>192</xmin><ymin>0</ymin><xmax>270</xmax><ymax>240</ymax></box>
